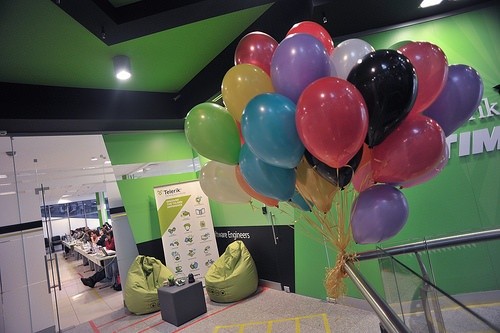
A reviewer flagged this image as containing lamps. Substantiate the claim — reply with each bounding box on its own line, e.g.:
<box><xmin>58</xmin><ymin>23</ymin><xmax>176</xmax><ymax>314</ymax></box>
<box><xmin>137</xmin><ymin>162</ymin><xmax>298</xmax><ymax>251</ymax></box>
<box><xmin>113</xmin><ymin>54</ymin><xmax>132</xmax><ymax>80</ymax></box>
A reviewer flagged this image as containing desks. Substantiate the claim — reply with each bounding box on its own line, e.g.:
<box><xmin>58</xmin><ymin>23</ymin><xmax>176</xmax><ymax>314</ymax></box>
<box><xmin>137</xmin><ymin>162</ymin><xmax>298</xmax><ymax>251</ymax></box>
<box><xmin>157</xmin><ymin>279</ymin><xmax>208</xmax><ymax>328</ymax></box>
<box><xmin>62</xmin><ymin>237</ymin><xmax>122</xmax><ymax>292</ymax></box>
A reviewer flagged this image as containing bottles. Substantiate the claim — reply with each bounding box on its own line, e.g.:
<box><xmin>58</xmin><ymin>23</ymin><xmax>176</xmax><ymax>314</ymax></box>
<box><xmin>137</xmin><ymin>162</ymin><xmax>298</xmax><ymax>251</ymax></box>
<box><xmin>174</xmin><ymin>266</ymin><xmax>187</xmax><ymax>286</ymax></box>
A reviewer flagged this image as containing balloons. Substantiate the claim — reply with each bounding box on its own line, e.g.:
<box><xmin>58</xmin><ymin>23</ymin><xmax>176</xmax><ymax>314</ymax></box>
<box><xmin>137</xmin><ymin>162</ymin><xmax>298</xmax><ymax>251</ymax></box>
<box><xmin>287</xmin><ymin>22</ymin><xmax>334</xmax><ymax>55</ymax></box>
<box><xmin>185</xmin><ymin>103</ymin><xmax>241</xmax><ymax>165</ymax></box>
<box><xmin>234</xmin><ymin>32</ymin><xmax>277</xmax><ymax>74</ymax></box>
<box><xmin>222</xmin><ymin>33</ymin><xmax>449</xmax><ymax>214</ymax></box>
<box><xmin>351</xmin><ymin>184</ymin><xmax>409</xmax><ymax>244</ymax></box>
<box><xmin>199</xmin><ymin>161</ymin><xmax>252</xmax><ymax>203</ymax></box>
<box><xmin>424</xmin><ymin>64</ymin><xmax>485</xmax><ymax>137</ymax></box>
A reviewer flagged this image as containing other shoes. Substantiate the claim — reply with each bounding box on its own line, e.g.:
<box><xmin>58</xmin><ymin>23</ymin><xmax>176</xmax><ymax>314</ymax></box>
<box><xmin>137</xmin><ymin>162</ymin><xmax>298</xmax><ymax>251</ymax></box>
<box><xmin>81</xmin><ymin>277</ymin><xmax>94</xmax><ymax>288</ymax></box>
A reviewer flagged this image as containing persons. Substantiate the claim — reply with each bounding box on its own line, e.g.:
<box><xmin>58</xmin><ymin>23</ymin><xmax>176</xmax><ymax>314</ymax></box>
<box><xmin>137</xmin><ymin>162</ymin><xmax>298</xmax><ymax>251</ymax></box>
<box><xmin>65</xmin><ymin>222</ymin><xmax>115</xmax><ymax>288</ymax></box>
